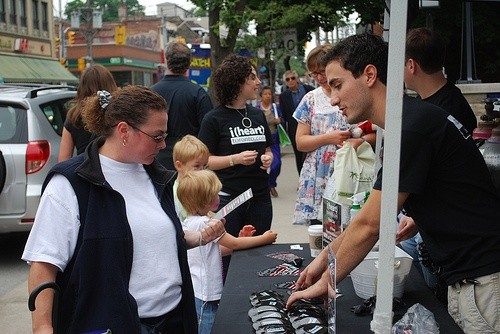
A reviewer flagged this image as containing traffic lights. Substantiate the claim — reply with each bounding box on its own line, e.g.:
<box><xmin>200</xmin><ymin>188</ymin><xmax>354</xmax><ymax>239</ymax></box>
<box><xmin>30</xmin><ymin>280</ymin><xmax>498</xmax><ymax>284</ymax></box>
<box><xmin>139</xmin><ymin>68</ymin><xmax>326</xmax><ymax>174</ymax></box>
<box><xmin>60</xmin><ymin>58</ymin><xmax>67</xmax><ymax>68</ymax></box>
<box><xmin>77</xmin><ymin>57</ymin><xmax>85</xmax><ymax>73</ymax></box>
<box><xmin>55</xmin><ymin>37</ymin><xmax>60</xmax><ymax>52</ymax></box>
<box><xmin>68</xmin><ymin>31</ymin><xmax>76</xmax><ymax>47</ymax></box>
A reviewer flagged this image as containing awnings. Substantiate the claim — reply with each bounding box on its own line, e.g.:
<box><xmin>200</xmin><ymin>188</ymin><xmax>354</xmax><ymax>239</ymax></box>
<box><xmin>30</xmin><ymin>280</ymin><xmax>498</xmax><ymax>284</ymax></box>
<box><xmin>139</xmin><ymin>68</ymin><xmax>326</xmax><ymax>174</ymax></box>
<box><xmin>0</xmin><ymin>55</ymin><xmax>80</xmax><ymax>85</ymax></box>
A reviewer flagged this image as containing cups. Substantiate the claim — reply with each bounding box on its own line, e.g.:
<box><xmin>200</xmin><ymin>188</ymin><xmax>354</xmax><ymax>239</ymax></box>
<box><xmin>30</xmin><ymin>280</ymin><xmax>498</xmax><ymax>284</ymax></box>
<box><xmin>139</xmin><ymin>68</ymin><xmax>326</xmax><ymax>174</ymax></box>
<box><xmin>307</xmin><ymin>224</ymin><xmax>325</xmax><ymax>258</ymax></box>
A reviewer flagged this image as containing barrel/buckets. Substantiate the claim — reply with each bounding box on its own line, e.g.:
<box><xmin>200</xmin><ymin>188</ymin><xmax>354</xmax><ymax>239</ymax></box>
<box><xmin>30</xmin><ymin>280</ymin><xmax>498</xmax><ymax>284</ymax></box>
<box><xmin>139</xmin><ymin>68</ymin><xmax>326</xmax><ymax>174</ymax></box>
<box><xmin>350</xmin><ymin>245</ymin><xmax>414</xmax><ymax>299</ymax></box>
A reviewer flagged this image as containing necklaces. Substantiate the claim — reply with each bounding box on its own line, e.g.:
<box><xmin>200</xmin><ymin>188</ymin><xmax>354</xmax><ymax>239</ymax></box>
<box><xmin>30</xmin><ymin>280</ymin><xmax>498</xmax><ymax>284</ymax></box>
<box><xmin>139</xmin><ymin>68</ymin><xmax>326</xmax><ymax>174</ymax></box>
<box><xmin>233</xmin><ymin>104</ymin><xmax>252</xmax><ymax>129</ymax></box>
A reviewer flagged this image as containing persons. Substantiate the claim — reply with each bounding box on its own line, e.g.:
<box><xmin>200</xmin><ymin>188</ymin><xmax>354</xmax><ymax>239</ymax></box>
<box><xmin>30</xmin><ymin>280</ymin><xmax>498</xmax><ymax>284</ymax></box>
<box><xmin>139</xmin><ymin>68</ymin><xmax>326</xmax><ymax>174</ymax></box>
<box><xmin>286</xmin><ymin>31</ymin><xmax>500</xmax><ymax>334</ymax></box>
<box><xmin>196</xmin><ymin>53</ymin><xmax>274</xmax><ymax>286</ymax></box>
<box><xmin>177</xmin><ymin>169</ymin><xmax>278</xmax><ymax>334</ymax></box>
<box><xmin>279</xmin><ymin>69</ymin><xmax>316</xmax><ymax>177</ymax></box>
<box><xmin>21</xmin><ymin>85</ymin><xmax>225</xmax><ymax>334</ymax></box>
<box><xmin>148</xmin><ymin>42</ymin><xmax>214</xmax><ymax>171</ymax></box>
<box><xmin>58</xmin><ymin>64</ymin><xmax>116</xmax><ymax>162</ymax></box>
<box><xmin>256</xmin><ymin>86</ymin><xmax>284</xmax><ymax>198</ymax></box>
<box><xmin>174</xmin><ymin>134</ymin><xmax>227</xmax><ymax>225</ymax></box>
<box><xmin>396</xmin><ymin>28</ymin><xmax>477</xmax><ymax>334</ymax></box>
<box><xmin>291</xmin><ymin>44</ymin><xmax>375</xmax><ymax>230</ymax></box>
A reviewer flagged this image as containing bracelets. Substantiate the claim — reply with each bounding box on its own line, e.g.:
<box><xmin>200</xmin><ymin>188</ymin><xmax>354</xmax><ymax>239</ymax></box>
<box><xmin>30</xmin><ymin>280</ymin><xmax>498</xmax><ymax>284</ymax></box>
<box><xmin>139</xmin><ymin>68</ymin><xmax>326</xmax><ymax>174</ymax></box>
<box><xmin>361</xmin><ymin>137</ymin><xmax>366</xmax><ymax>142</ymax></box>
<box><xmin>229</xmin><ymin>157</ymin><xmax>234</xmax><ymax>167</ymax></box>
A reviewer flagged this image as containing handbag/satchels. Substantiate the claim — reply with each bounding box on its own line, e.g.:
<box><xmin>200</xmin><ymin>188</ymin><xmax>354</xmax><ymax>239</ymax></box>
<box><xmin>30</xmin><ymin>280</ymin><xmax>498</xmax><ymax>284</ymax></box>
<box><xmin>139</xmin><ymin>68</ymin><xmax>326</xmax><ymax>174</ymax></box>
<box><xmin>272</xmin><ymin>103</ymin><xmax>292</xmax><ymax>149</ymax></box>
<box><xmin>322</xmin><ymin>141</ymin><xmax>375</xmax><ymax>207</ymax></box>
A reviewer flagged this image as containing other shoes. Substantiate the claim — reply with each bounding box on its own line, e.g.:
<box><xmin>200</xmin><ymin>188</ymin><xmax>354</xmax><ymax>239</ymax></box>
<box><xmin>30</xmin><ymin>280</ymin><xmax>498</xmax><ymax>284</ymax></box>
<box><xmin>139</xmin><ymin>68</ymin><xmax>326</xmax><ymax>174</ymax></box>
<box><xmin>269</xmin><ymin>186</ymin><xmax>278</xmax><ymax>197</ymax></box>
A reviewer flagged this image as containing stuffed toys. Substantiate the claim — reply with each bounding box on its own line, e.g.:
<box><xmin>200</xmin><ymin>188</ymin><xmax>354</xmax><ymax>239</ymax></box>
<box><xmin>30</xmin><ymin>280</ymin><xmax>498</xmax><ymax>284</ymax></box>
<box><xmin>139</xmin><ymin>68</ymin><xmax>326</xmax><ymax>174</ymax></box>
<box><xmin>352</xmin><ymin>120</ymin><xmax>377</xmax><ymax>138</ymax></box>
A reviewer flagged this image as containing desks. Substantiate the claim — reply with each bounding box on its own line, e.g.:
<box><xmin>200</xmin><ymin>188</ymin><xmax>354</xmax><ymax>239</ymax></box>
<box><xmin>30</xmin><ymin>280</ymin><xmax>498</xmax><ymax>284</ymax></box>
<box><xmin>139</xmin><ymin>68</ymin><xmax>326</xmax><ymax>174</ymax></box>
<box><xmin>210</xmin><ymin>242</ymin><xmax>466</xmax><ymax>334</ymax></box>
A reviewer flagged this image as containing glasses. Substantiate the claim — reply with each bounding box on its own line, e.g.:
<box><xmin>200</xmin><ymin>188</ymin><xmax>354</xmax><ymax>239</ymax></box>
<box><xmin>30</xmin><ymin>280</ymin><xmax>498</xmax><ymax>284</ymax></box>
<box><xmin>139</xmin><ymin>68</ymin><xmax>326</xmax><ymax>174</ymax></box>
<box><xmin>111</xmin><ymin>121</ymin><xmax>169</xmax><ymax>143</ymax></box>
<box><xmin>285</xmin><ymin>77</ymin><xmax>298</xmax><ymax>81</ymax></box>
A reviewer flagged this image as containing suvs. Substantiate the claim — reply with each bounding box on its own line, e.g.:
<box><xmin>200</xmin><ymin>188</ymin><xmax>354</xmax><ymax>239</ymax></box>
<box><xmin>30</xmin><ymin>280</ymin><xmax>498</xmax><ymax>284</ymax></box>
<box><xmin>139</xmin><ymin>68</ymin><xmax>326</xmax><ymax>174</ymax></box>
<box><xmin>0</xmin><ymin>82</ymin><xmax>79</xmax><ymax>235</ymax></box>
